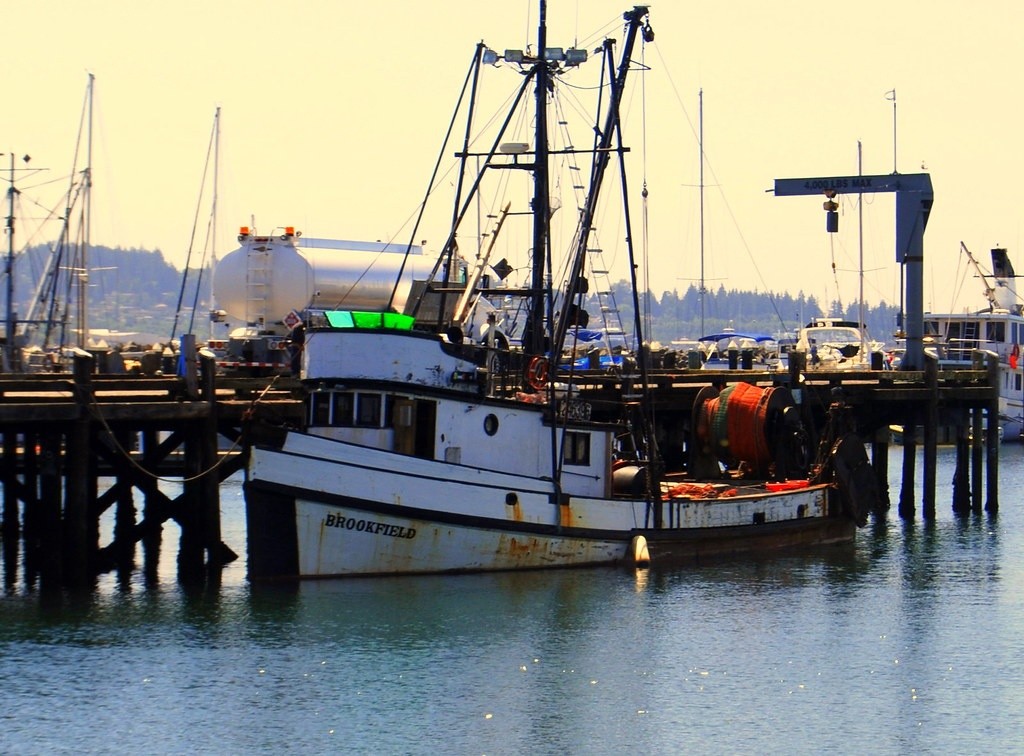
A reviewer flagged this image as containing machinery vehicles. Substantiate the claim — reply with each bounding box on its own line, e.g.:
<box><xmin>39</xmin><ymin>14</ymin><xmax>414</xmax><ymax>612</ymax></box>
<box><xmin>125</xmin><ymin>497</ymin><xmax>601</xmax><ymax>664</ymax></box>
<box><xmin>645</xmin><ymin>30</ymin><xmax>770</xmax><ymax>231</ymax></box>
<box><xmin>207</xmin><ymin>226</ymin><xmax>511</xmax><ymax>400</ymax></box>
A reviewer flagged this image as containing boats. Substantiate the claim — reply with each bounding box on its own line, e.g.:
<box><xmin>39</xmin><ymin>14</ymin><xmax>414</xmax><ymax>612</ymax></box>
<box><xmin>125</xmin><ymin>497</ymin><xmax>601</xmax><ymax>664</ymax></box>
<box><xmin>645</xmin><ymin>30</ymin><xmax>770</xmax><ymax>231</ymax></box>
<box><xmin>699</xmin><ymin>332</ymin><xmax>797</xmax><ymax>370</ymax></box>
<box><xmin>796</xmin><ymin>317</ymin><xmax>886</xmax><ymax>369</ymax></box>
<box><xmin>893</xmin><ymin>241</ymin><xmax>1024</xmax><ymax>442</ymax></box>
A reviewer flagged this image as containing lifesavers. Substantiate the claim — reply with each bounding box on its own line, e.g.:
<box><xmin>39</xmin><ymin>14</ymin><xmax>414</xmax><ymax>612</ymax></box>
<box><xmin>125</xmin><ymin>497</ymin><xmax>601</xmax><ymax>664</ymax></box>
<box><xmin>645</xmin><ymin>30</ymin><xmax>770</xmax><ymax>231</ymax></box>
<box><xmin>528</xmin><ymin>356</ymin><xmax>549</xmax><ymax>390</ymax></box>
<box><xmin>42</xmin><ymin>352</ymin><xmax>55</xmax><ymax>372</ymax></box>
<box><xmin>1013</xmin><ymin>344</ymin><xmax>1020</xmax><ymax>358</ymax></box>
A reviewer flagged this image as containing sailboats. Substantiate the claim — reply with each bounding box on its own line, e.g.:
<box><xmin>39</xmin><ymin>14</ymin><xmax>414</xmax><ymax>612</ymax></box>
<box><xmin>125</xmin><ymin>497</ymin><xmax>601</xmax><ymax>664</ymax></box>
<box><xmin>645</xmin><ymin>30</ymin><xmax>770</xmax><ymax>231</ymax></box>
<box><xmin>248</xmin><ymin>0</ymin><xmax>876</xmax><ymax>577</ymax></box>
